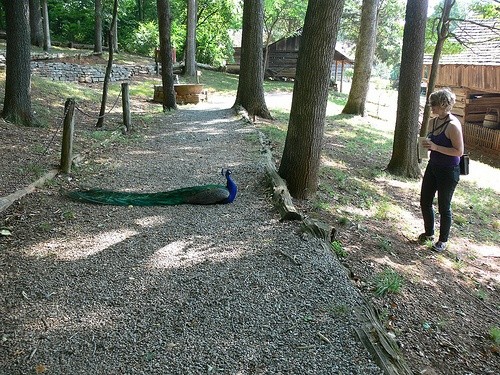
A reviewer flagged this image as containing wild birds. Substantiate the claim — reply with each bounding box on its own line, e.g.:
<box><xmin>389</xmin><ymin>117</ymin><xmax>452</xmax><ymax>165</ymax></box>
<box><xmin>62</xmin><ymin>168</ymin><xmax>238</xmax><ymax>207</ymax></box>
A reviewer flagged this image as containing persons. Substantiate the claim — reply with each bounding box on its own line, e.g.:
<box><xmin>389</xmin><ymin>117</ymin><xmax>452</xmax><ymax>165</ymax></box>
<box><xmin>418</xmin><ymin>89</ymin><xmax>464</xmax><ymax>250</ymax></box>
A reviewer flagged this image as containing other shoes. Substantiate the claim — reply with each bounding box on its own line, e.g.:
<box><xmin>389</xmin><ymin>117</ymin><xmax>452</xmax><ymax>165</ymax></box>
<box><xmin>413</xmin><ymin>233</ymin><xmax>435</xmax><ymax>243</ymax></box>
<box><xmin>432</xmin><ymin>240</ymin><xmax>447</xmax><ymax>252</ymax></box>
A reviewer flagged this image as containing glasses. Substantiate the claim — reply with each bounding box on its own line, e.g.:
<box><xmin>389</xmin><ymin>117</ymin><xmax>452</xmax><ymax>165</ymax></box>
<box><xmin>429</xmin><ymin>102</ymin><xmax>441</xmax><ymax>106</ymax></box>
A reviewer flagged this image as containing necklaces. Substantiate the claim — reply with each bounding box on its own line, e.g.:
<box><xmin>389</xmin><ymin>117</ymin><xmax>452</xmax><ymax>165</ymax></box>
<box><xmin>435</xmin><ymin>114</ymin><xmax>449</xmax><ymax>134</ymax></box>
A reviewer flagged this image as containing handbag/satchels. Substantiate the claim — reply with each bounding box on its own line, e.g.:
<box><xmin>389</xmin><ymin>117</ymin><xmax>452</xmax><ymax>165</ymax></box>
<box><xmin>460</xmin><ymin>154</ymin><xmax>470</xmax><ymax>175</ymax></box>
